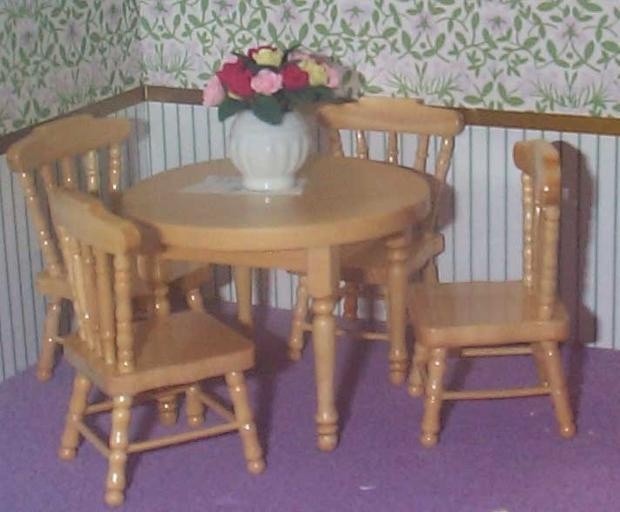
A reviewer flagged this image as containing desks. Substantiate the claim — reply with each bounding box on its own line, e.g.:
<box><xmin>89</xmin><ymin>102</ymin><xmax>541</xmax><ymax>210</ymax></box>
<box><xmin>123</xmin><ymin>158</ymin><xmax>433</xmax><ymax>450</ymax></box>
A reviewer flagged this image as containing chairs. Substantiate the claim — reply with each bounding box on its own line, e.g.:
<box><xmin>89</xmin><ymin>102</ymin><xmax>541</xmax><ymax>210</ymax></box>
<box><xmin>284</xmin><ymin>94</ymin><xmax>463</xmax><ymax>359</ymax></box>
<box><xmin>408</xmin><ymin>140</ymin><xmax>581</xmax><ymax>446</ymax></box>
<box><xmin>49</xmin><ymin>187</ymin><xmax>269</xmax><ymax>508</ymax></box>
<box><xmin>9</xmin><ymin>111</ymin><xmax>212</xmax><ymax>382</ymax></box>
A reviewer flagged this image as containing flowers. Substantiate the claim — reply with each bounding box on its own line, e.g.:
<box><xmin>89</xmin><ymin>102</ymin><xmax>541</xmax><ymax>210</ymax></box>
<box><xmin>203</xmin><ymin>42</ymin><xmax>344</xmax><ymax>125</ymax></box>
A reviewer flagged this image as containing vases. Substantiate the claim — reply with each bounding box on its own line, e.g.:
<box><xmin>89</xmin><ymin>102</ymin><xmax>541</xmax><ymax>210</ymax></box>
<box><xmin>229</xmin><ymin>110</ymin><xmax>310</xmax><ymax>192</ymax></box>
